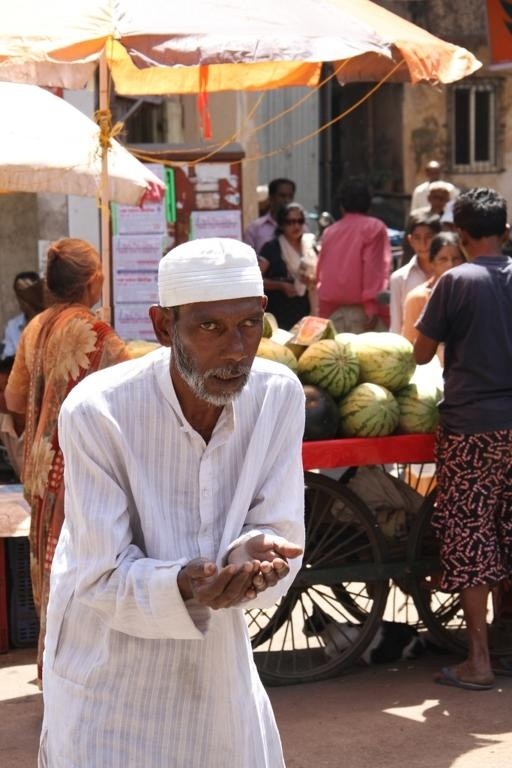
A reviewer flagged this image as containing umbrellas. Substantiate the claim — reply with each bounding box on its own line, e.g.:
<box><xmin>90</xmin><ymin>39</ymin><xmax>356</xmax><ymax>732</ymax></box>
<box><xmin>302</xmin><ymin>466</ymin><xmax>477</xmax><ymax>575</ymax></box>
<box><xmin>1</xmin><ymin>78</ymin><xmax>168</xmax><ymax>207</ymax></box>
<box><xmin>2</xmin><ymin>0</ymin><xmax>487</xmax><ymax>326</ymax></box>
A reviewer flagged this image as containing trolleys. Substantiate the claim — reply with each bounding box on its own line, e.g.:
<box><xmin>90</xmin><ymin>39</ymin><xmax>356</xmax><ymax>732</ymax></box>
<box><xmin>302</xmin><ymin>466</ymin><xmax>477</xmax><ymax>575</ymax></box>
<box><xmin>244</xmin><ymin>430</ymin><xmax>511</xmax><ymax>685</ymax></box>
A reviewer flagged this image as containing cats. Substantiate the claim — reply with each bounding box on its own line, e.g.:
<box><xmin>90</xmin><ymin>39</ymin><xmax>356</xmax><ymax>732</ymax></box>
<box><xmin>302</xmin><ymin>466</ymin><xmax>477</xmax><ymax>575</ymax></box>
<box><xmin>301</xmin><ymin>603</ymin><xmax>452</xmax><ymax>665</ymax></box>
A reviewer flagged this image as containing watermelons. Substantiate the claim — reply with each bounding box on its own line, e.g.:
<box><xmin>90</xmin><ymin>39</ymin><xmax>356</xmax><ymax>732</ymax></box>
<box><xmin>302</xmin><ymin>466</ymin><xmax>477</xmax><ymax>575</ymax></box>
<box><xmin>255</xmin><ymin>315</ymin><xmax>444</xmax><ymax>442</ymax></box>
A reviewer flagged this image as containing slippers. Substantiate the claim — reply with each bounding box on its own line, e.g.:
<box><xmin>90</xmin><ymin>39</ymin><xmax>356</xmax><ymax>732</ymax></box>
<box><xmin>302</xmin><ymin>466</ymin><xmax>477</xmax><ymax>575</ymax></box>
<box><xmin>489</xmin><ymin>661</ymin><xmax>511</xmax><ymax>677</ymax></box>
<box><xmin>434</xmin><ymin>665</ymin><xmax>496</xmax><ymax>691</ymax></box>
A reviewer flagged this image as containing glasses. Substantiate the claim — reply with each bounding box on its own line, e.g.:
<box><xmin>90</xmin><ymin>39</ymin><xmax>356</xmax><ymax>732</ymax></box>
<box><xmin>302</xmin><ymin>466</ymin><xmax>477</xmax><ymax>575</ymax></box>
<box><xmin>282</xmin><ymin>217</ymin><xmax>304</xmax><ymax>226</ymax></box>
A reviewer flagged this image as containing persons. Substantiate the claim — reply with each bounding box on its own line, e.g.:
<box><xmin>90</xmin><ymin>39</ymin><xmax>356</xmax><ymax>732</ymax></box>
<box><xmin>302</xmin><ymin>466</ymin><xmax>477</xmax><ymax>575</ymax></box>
<box><xmin>31</xmin><ymin>233</ymin><xmax>309</xmax><ymax>766</ymax></box>
<box><xmin>1</xmin><ymin>267</ymin><xmax>41</xmax><ymax>355</ymax></box>
<box><xmin>241</xmin><ymin>178</ymin><xmax>314</xmax><ymax>331</ymax></box>
<box><xmin>4</xmin><ymin>234</ymin><xmax>131</xmax><ymax>686</ymax></box>
<box><xmin>315</xmin><ymin>176</ymin><xmax>392</xmax><ymax>334</ymax></box>
<box><xmin>391</xmin><ymin>161</ymin><xmax>463</xmax><ymax>368</ymax></box>
<box><xmin>411</xmin><ymin>185</ymin><xmax>512</xmax><ymax>689</ymax></box>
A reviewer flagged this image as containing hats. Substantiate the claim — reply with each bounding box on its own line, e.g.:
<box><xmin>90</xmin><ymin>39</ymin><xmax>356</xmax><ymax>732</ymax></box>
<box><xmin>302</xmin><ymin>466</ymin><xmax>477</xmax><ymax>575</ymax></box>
<box><xmin>153</xmin><ymin>238</ymin><xmax>270</xmax><ymax>310</ymax></box>
